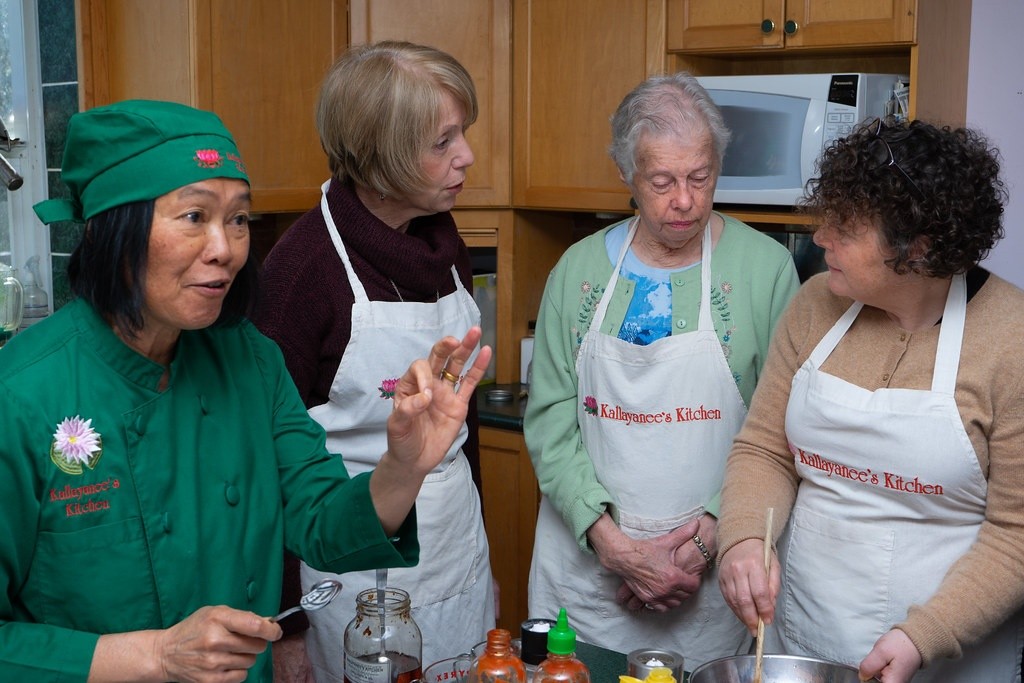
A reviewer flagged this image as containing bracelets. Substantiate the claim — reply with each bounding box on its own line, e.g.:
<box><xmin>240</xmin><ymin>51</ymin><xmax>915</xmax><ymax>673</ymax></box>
<box><xmin>691</xmin><ymin>534</ymin><xmax>714</xmax><ymax>570</ymax></box>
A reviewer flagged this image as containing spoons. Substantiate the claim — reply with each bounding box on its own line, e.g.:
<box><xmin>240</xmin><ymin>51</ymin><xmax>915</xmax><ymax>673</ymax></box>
<box><xmin>269</xmin><ymin>579</ymin><xmax>342</xmax><ymax>623</ymax></box>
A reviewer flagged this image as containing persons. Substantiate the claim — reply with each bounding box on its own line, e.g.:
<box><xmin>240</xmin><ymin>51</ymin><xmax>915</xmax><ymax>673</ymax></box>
<box><xmin>713</xmin><ymin>119</ymin><xmax>1024</xmax><ymax>683</ymax></box>
<box><xmin>235</xmin><ymin>41</ymin><xmax>492</xmax><ymax>683</ymax></box>
<box><xmin>522</xmin><ymin>73</ymin><xmax>802</xmax><ymax>669</ymax></box>
<box><xmin>0</xmin><ymin>99</ymin><xmax>494</xmax><ymax>683</ymax></box>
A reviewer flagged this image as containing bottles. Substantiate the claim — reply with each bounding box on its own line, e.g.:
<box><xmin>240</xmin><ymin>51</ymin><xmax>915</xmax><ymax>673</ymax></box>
<box><xmin>532</xmin><ymin>607</ymin><xmax>592</xmax><ymax>683</ymax></box>
<box><xmin>519</xmin><ymin>320</ymin><xmax>537</xmax><ymax>398</ymax></box>
<box><xmin>467</xmin><ymin>629</ymin><xmax>527</xmax><ymax>683</ymax></box>
<box><xmin>344</xmin><ymin>587</ymin><xmax>422</xmax><ymax>683</ymax></box>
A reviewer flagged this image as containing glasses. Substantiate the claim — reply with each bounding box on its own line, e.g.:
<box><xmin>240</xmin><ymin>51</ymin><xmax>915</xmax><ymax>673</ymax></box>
<box><xmin>862</xmin><ymin>116</ymin><xmax>933</xmax><ymax>212</ymax></box>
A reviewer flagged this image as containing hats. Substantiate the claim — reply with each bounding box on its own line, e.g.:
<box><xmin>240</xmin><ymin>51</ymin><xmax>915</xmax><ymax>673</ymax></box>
<box><xmin>32</xmin><ymin>99</ymin><xmax>251</xmax><ymax>225</ymax></box>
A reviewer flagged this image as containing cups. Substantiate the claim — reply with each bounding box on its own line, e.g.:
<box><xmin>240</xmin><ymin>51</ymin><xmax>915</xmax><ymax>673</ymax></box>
<box><xmin>408</xmin><ymin>639</ymin><xmax>519</xmax><ymax>683</ymax></box>
<box><xmin>521</xmin><ymin>619</ymin><xmax>557</xmax><ymax>665</ymax></box>
<box><xmin>0</xmin><ymin>262</ymin><xmax>24</xmax><ymax>335</ymax></box>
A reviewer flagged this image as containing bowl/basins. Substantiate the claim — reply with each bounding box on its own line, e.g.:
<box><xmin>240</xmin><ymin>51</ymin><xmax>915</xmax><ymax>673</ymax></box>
<box><xmin>688</xmin><ymin>653</ymin><xmax>882</xmax><ymax>683</ymax></box>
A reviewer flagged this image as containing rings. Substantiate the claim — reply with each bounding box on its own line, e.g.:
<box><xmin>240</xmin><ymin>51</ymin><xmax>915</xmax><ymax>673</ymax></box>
<box><xmin>441</xmin><ymin>368</ymin><xmax>460</xmax><ymax>383</ymax></box>
<box><xmin>644</xmin><ymin>603</ymin><xmax>654</xmax><ymax>610</ymax></box>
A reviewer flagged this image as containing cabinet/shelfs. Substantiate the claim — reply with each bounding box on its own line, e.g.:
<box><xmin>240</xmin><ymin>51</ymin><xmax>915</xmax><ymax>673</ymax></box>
<box><xmin>75</xmin><ymin>0</ymin><xmax>972</xmax><ymax>635</ymax></box>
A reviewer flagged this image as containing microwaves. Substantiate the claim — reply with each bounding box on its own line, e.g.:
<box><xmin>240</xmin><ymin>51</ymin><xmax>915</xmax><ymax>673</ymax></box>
<box><xmin>692</xmin><ymin>72</ymin><xmax>910</xmax><ymax>206</ymax></box>
